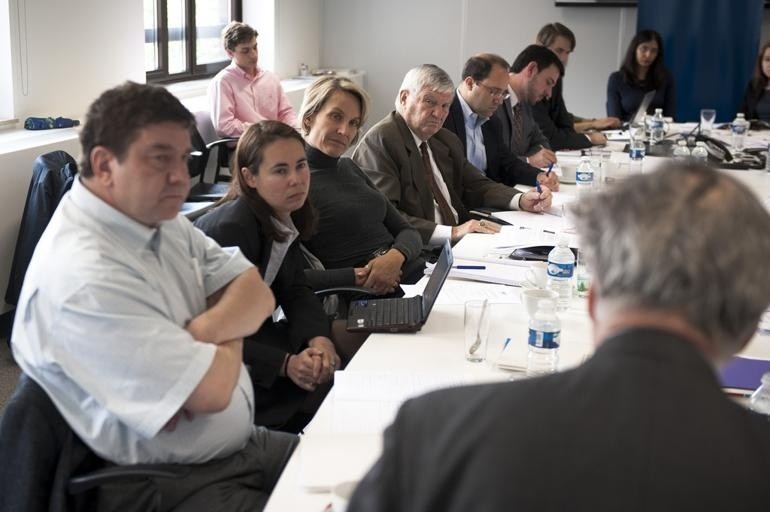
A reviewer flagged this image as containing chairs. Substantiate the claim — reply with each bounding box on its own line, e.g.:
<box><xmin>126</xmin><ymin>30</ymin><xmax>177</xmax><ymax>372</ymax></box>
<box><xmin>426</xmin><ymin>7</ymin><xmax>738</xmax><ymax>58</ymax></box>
<box><xmin>0</xmin><ymin>373</ymin><xmax>181</xmax><ymax>510</ymax></box>
<box><xmin>187</xmin><ymin>125</ymin><xmax>243</xmax><ymax>201</ymax></box>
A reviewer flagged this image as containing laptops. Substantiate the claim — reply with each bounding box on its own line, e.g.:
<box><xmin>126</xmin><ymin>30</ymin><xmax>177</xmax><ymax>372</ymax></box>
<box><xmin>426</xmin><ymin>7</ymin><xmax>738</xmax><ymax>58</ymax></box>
<box><xmin>594</xmin><ymin>90</ymin><xmax>658</xmax><ymax>131</ymax></box>
<box><xmin>344</xmin><ymin>239</ymin><xmax>454</xmax><ymax>334</ymax></box>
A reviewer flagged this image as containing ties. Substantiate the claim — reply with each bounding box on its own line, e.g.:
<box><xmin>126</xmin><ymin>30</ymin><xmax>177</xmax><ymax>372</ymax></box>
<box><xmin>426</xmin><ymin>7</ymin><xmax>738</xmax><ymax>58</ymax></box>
<box><xmin>419</xmin><ymin>142</ymin><xmax>457</xmax><ymax>227</ymax></box>
<box><xmin>512</xmin><ymin>100</ymin><xmax>526</xmax><ymax>158</ymax></box>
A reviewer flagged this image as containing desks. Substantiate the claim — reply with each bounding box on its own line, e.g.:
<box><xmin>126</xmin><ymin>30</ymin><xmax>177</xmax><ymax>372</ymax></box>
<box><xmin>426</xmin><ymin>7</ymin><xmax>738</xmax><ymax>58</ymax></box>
<box><xmin>262</xmin><ymin>119</ymin><xmax>770</xmax><ymax>512</ymax></box>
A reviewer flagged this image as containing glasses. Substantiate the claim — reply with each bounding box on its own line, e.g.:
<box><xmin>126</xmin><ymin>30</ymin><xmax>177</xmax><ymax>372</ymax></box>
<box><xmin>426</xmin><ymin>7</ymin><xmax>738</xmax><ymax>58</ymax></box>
<box><xmin>474</xmin><ymin>79</ymin><xmax>511</xmax><ymax>100</ymax></box>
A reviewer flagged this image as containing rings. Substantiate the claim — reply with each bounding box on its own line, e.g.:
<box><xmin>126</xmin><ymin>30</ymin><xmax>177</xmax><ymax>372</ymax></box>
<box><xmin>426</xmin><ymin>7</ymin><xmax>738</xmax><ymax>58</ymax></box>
<box><xmin>479</xmin><ymin>221</ymin><xmax>487</xmax><ymax>227</ymax></box>
<box><xmin>329</xmin><ymin>361</ymin><xmax>336</xmax><ymax>367</ymax></box>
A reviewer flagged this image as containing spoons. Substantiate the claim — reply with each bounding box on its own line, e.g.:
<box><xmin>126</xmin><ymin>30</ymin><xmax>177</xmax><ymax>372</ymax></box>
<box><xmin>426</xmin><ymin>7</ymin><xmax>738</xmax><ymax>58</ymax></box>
<box><xmin>469</xmin><ymin>299</ymin><xmax>486</xmax><ymax>353</ymax></box>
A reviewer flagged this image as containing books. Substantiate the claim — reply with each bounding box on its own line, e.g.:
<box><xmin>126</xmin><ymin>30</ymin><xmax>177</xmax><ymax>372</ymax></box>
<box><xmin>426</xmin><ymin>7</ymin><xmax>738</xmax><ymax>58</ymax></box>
<box><xmin>423</xmin><ymin>232</ymin><xmax>552</xmax><ymax>288</ymax></box>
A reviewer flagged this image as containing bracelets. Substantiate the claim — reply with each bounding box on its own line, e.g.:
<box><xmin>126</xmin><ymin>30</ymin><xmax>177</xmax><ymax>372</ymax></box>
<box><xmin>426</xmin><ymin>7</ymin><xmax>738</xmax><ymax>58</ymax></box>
<box><xmin>527</xmin><ymin>157</ymin><xmax>530</xmax><ymax>164</ymax></box>
<box><xmin>285</xmin><ymin>353</ymin><xmax>294</xmax><ymax>379</ymax></box>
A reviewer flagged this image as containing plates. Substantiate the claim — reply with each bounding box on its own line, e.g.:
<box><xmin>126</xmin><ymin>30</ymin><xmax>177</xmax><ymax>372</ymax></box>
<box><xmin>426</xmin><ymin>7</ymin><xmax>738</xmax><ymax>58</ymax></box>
<box><xmin>556</xmin><ymin>176</ymin><xmax>576</xmax><ymax>185</ymax></box>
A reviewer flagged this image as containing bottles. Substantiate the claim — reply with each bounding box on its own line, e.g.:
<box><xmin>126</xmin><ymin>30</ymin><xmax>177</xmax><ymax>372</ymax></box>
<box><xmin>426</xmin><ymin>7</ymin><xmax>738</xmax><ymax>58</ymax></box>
<box><xmin>629</xmin><ymin>136</ymin><xmax>646</xmax><ymax>175</ymax></box>
<box><xmin>767</xmin><ymin>141</ymin><xmax>770</xmax><ymax>172</ymax></box>
<box><xmin>692</xmin><ymin>141</ymin><xmax>708</xmax><ymax>166</ymax></box>
<box><xmin>672</xmin><ymin>141</ymin><xmax>690</xmax><ymax>165</ymax></box>
<box><xmin>547</xmin><ymin>237</ymin><xmax>575</xmax><ymax>311</ymax></box>
<box><xmin>650</xmin><ymin>109</ymin><xmax>663</xmax><ymax>145</ymax></box>
<box><xmin>527</xmin><ymin>300</ymin><xmax>561</xmax><ymax>378</ymax></box>
<box><xmin>749</xmin><ymin>371</ymin><xmax>770</xmax><ymax>419</ymax></box>
<box><xmin>732</xmin><ymin>113</ymin><xmax>749</xmax><ymax>151</ymax></box>
<box><xmin>575</xmin><ymin>156</ymin><xmax>594</xmax><ymax>186</ymax></box>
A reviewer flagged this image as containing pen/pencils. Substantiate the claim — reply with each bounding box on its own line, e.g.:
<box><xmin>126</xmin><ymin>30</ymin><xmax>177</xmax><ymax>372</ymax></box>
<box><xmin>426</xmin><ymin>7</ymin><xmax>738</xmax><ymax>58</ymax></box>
<box><xmin>533</xmin><ymin>177</ymin><xmax>547</xmax><ymax>213</ymax></box>
<box><xmin>538</xmin><ymin>143</ymin><xmax>560</xmax><ymax>169</ymax></box>
<box><xmin>451</xmin><ymin>264</ymin><xmax>486</xmax><ymax>271</ymax></box>
<box><xmin>545</xmin><ymin>159</ymin><xmax>555</xmax><ymax>179</ymax></box>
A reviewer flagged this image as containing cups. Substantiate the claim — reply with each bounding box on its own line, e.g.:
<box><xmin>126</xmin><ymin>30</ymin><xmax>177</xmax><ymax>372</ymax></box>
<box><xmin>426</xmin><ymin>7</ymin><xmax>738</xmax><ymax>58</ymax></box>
<box><xmin>600</xmin><ymin>155</ymin><xmax>620</xmax><ymax>184</ymax></box>
<box><xmin>464</xmin><ymin>300</ymin><xmax>491</xmax><ymax>363</ymax></box>
<box><xmin>700</xmin><ymin>109</ymin><xmax>715</xmax><ymax>129</ymax></box>
<box><xmin>630</xmin><ymin>116</ymin><xmax>646</xmax><ymax>139</ymax></box>
<box><xmin>521</xmin><ymin>289</ymin><xmax>559</xmax><ymax>317</ymax></box>
<box><xmin>560</xmin><ymin>165</ymin><xmax>578</xmax><ymax>180</ymax></box>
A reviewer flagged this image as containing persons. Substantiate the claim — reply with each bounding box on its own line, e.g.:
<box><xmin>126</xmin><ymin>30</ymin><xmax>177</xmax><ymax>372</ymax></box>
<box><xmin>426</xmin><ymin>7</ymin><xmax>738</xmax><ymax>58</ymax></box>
<box><xmin>351</xmin><ymin>64</ymin><xmax>553</xmax><ymax>264</ymax></box>
<box><xmin>531</xmin><ymin>23</ymin><xmax>607</xmax><ymax>152</ymax></box>
<box><xmin>442</xmin><ymin>53</ymin><xmax>559</xmax><ymax>193</ymax></box>
<box><xmin>606</xmin><ymin>29</ymin><xmax>677</xmax><ymax>125</ymax></box>
<box><xmin>737</xmin><ymin>43</ymin><xmax>770</xmax><ymax>126</ymax></box>
<box><xmin>493</xmin><ymin>45</ymin><xmax>565</xmax><ymax>169</ymax></box>
<box><xmin>208</xmin><ymin>22</ymin><xmax>298</xmax><ymax>178</ymax></box>
<box><xmin>191</xmin><ymin>120</ymin><xmax>371</xmax><ymax>434</ymax></box>
<box><xmin>298</xmin><ymin>73</ymin><xmax>426</xmax><ymax>317</ymax></box>
<box><xmin>573</xmin><ymin>115</ymin><xmax>620</xmax><ymax>132</ymax></box>
<box><xmin>345</xmin><ymin>159</ymin><xmax>770</xmax><ymax>512</ymax></box>
<box><xmin>9</xmin><ymin>79</ymin><xmax>301</xmax><ymax>511</ymax></box>
